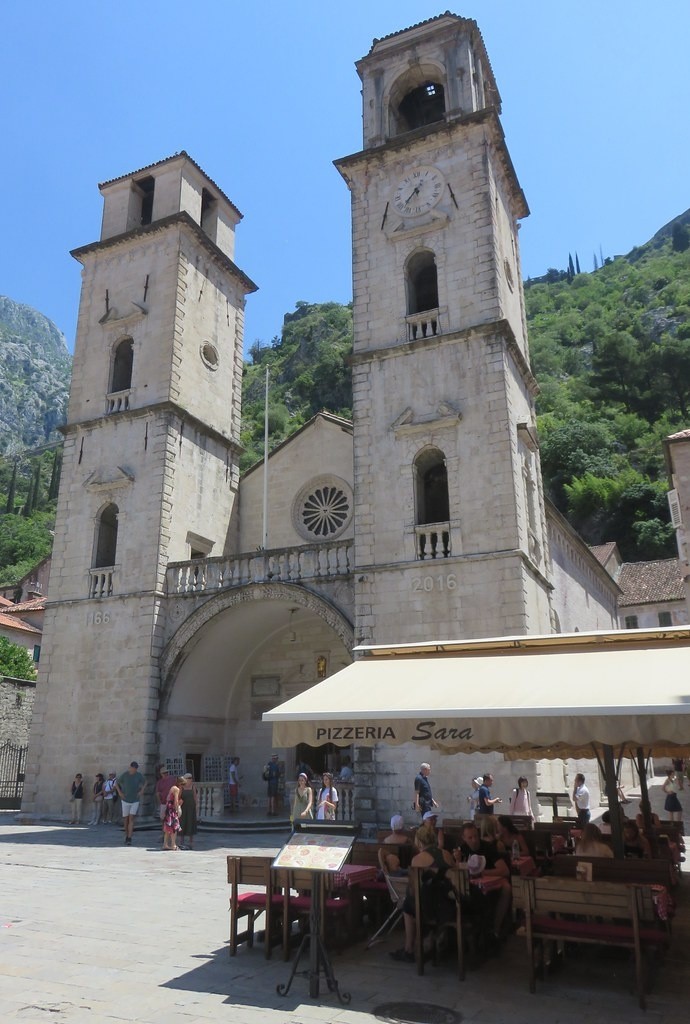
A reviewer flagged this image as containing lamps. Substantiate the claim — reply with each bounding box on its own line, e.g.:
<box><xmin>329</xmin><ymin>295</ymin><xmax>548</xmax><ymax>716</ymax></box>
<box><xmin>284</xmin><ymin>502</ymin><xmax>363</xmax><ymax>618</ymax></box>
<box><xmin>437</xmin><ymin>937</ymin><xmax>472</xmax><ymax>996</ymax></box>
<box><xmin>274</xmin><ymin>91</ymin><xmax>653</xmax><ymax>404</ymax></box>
<box><xmin>288</xmin><ymin>607</ymin><xmax>300</xmax><ymax>641</ymax></box>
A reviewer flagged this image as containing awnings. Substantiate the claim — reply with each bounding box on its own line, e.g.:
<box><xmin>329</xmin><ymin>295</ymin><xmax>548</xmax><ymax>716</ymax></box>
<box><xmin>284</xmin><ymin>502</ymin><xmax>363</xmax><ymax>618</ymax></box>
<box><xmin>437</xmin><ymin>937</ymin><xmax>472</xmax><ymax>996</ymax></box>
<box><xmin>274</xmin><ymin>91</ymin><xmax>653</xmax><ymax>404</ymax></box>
<box><xmin>262</xmin><ymin>626</ymin><xmax>690</xmax><ymax>749</ymax></box>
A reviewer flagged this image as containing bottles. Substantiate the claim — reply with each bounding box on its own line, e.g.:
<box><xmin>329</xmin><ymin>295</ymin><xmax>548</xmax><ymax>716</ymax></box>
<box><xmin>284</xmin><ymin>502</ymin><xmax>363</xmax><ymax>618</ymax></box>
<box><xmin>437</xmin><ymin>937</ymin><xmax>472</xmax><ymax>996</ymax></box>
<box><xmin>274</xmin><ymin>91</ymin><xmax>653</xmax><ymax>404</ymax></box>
<box><xmin>512</xmin><ymin>839</ymin><xmax>520</xmax><ymax>860</ymax></box>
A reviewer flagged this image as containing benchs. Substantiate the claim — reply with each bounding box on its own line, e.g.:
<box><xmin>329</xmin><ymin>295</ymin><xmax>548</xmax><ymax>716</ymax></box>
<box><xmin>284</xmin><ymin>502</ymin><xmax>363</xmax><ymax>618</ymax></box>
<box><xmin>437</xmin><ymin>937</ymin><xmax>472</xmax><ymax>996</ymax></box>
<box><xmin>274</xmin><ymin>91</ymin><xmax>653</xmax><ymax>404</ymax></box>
<box><xmin>227</xmin><ymin>820</ymin><xmax>684</xmax><ymax>1012</ymax></box>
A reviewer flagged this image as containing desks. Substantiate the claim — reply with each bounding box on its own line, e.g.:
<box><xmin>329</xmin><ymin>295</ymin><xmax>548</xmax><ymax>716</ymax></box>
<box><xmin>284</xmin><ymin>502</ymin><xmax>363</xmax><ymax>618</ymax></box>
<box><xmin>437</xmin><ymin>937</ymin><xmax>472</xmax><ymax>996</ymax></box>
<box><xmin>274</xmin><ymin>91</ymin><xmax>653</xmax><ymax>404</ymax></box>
<box><xmin>513</xmin><ymin>855</ymin><xmax>536</xmax><ymax>874</ymax></box>
<box><xmin>333</xmin><ymin>864</ymin><xmax>377</xmax><ymax>938</ymax></box>
<box><xmin>646</xmin><ymin>884</ymin><xmax>673</xmax><ymax>964</ymax></box>
<box><xmin>467</xmin><ymin>875</ymin><xmax>506</xmax><ymax>958</ymax></box>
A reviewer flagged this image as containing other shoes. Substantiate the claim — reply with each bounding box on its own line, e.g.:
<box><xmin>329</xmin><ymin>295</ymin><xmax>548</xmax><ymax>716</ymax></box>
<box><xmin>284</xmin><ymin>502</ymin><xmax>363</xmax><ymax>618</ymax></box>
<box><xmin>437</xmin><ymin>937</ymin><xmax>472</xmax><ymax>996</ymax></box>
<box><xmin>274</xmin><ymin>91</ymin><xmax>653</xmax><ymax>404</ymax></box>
<box><xmin>69</xmin><ymin>821</ymin><xmax>75</xmax><ymax>824</ymax></box>
<box><xmin>160</xmin><ymin>839</ymin><xmax>194</xmax><ymax>851</ymax></box>
<box><xmin>93</xmin><ymin>821</ymin><xmax>98</xmax><ymax>825</ymax></box>
<box><xmin>88</xmin><ymin>820</ymin><xmax>94</xmax><ymax>825</ymax></box>
<box><xmin>126</xmin><ymin>837</ymin><xmax>131</xmax><ymax>844</ymax></box>
<box><xmin>391</xmin><ymin>917</ymin><xmax>527</xmax><ymax>964</ymax></box>
<box><xmin>76</xmin><ymin>821</ymin><xmax>81</xmax><ymax>824</ymax></box>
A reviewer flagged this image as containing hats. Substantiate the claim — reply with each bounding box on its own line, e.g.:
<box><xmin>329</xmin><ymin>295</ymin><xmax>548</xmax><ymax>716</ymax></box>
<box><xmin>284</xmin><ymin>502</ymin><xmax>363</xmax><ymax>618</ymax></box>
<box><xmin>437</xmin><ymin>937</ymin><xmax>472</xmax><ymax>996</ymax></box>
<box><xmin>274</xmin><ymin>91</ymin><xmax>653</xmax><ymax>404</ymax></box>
<box><xmin>272</xmin><ymin>754</ymin><xmax>278</xmax><ymax>758</ymax></box>
<box><xmin>160</xmin><ymin>767</ymin><xmax>168</xmax><ymax>773</ymax></box>
<box><xmin>390</xmin><ymin>815</ymin><xmax>403</xmax><ymax>830</ymax></box>
<box><xmin>467</xmin><ymin>854</ymin><xmax>486</xmax><ymax>875</ymax></box>
<box><xmin>95</xmin><ymin>774</ymin><xmax>103</xmax><ymax>778</ymax></box>
<box><xmin>474</xmin><ymin>777</ymin><xmax>483</xmax><ymax>787</ymax></box>
<box><xmin>423</xmin><ymin>811</ymin><xmax>439</xmax><ymax>820</ymax></box>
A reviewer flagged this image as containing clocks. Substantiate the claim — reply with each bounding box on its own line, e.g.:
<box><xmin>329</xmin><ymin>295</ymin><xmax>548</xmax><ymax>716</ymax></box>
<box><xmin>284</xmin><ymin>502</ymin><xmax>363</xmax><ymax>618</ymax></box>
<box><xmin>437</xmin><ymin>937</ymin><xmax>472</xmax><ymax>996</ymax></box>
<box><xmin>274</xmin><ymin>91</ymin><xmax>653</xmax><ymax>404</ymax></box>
<box><xmin>392</xmin><ymin>165</ymin><xmax>445</xmax><ymax>219</ymax></box>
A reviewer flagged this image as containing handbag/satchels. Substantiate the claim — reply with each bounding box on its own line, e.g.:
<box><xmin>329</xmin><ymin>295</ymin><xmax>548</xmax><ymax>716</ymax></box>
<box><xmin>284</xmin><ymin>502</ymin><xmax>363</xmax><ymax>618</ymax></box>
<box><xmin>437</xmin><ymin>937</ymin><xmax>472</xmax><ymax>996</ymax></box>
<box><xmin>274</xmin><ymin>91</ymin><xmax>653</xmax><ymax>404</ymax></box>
<box><xmin>95</xmin><ymin>795</ymin><xmax>103</xmax><ymax>801</ymax></box>
<box><xmin>664</xmin><ymin>779</ymin><xmax>676</xmax><ymax>794</ymax></box>
<box><xmin>113</xmin><ymin>795</ymin><xmax>118</xmax><ymax>801</ymax></box>
<box><xmin>69</xmin><ymin>795</ymin><xmax>75</xmax><ymax>802</ymax></box>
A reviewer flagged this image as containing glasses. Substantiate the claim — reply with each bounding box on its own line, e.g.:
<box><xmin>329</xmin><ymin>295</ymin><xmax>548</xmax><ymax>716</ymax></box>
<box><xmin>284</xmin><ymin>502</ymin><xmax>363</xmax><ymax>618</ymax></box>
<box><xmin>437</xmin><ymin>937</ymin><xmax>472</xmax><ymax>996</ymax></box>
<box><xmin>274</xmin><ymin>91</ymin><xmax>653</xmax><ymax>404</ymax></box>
<box><xmin>186</xmin><ymin>777</ymin><xmax>193</xmax><ymax>779</ymax></box>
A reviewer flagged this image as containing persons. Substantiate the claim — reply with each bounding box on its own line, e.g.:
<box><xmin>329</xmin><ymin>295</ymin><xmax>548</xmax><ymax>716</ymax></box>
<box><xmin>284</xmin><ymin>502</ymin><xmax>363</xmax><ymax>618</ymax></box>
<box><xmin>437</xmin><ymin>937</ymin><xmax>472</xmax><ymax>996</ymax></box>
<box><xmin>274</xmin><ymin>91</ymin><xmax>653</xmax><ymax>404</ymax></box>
<box><xmin>294</xmin><ymin>756</ymin><xmax>356</xmax><ymax>782</ymax></box>
<box><xmin>315</xmin><ymin>772</ymin><xmax>340</xmax><ymax>821</ymax></box>
<box><xmin>573</xmin><ymin>774</ymin><xmax>591</xmax><ymax>825</ymax></box>
<box><xmin>377</xmin><ymin>811</ymin><xmax>686</xmax><ymax>970</ymax></box>
<box><xmin>510</xmin><ymin>776</ymin><xmax>535</xmax><ymax>828</ymax></box>
<box><xmin>672</xmin><ymin>753</ymin><xmax>685</xmax><ymax>791</ymax></box>
<box><xmin>155</xmin><ymin>766</ymin><xmax>199</xmax><ymax>851</ymax></box>
<box><xmin>663</xmin><ymin>769</ymin><xmax>683</xmax><ymax>822</ymax></box>
<box><xmin>262</xmin><ymin>754</ymin><xmax>282</xmax><ymax>816</ymax></box>
<box><xmin>414</xmin><ymin>763</ymin><xmax>439</xmax><ymax>819</ymax></box>
<box><xmin>229</xmin><ymin>757</ymin><xmax>242</xmax><ymax>812</ymax></box>
<box><xmin>468</xmin><ymin>773</ymin><xmax>503</xmax><ymax>820</ymax></box>
<box><xmin>115</xmin><ymin>762</ymin><xmax>147</xmax><ymax>846</ymax></box>
<box><xmin>616</xmin><ymin>776</ymin><xmax>632</xmax><ymax>805</ymax></box>
<box><xmin>69</xmin><ymin>773</ymin><xmax>118</xmax><ymax>825</ymax></box>
<box><xmin>292</xmin><ymin>773</ymin><xmax>314</xmax><ymax>831</ymax></box>
